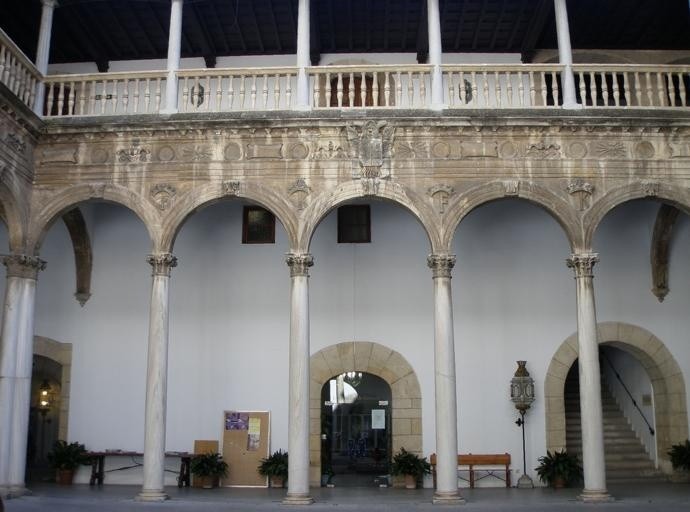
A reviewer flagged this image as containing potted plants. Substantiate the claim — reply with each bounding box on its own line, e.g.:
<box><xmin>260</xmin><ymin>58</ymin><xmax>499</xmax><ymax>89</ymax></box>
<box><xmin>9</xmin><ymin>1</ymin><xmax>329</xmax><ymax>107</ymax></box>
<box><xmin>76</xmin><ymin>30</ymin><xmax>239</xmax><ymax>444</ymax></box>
<box><xmin>190</xmin><ymin>453</ymin><xmax>229</xmax><ymax>488</ymax></box>
<box><xmin>257</xmin><ymin>448</ymin><xmax>288</xmax><ymax>488</ymax></box>
<box><xmin>535</xmin><ymin>448</ymin><xmax>584</xmax><ymax>488</ymax></box>
<box><xmin>389</xmin><ymin>447</ymin><xmax>433</xmax><ymax>488</ymax></box>
<box><xmin>46</xmin><ymin>440</ymin><xmax>88</xmax><ymax>486</ymax></box>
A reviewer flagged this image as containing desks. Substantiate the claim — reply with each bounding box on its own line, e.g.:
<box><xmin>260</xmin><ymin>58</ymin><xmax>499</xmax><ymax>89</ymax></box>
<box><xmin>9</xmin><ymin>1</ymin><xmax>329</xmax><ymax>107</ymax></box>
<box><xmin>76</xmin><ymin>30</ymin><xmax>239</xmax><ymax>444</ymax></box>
<box><xmin>80</xmin><ymin>451</ymin><xmax>200</xmax><ymax>487</ymax></box>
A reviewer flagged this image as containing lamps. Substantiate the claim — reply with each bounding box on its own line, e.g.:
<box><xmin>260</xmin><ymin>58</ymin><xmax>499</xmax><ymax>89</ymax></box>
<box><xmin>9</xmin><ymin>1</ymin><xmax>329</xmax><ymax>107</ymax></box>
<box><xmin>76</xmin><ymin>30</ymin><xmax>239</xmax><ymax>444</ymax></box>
<box><xmin>511</xmin><ymin>361</ymin><xmax>535</xmax><ymax>489</ymax></box>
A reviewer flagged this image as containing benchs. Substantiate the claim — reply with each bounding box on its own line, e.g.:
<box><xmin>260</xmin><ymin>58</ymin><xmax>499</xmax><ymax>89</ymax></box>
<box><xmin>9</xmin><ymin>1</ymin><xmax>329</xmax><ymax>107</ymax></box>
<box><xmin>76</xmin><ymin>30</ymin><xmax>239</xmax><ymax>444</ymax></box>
<box><xmin>430</xmin><ymin>453</ymin><xmax>513</xmax><ymax>488</ymax></box>
